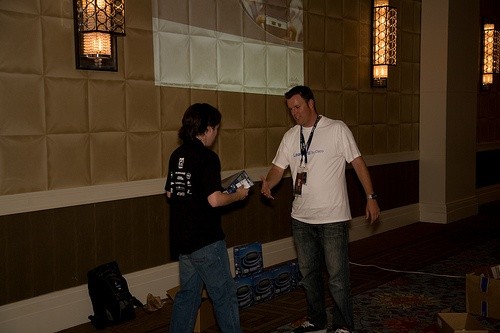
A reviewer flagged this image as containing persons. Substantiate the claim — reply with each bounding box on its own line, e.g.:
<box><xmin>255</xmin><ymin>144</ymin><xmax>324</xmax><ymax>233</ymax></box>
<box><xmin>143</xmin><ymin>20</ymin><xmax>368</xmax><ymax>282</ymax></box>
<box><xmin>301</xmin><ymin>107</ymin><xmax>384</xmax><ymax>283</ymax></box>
<box><xmin>165</xmin><ymin>102</ymin><xmax>249</xmax><ymax>333</ymax></box>
<box><xmin>260</xmin><ymin>85</ymin><xmax>380</xmax><ymax>333</ymax></box>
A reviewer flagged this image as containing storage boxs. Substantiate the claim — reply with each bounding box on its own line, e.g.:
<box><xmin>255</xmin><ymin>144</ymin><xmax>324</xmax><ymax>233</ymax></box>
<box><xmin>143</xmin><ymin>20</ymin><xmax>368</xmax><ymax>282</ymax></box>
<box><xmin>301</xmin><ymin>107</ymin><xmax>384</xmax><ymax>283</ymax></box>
<box><xmin>166</xmin><ymin>245</ymin><xmax>301</xmax><ymax>332</ymax></box>
<box><xmin>436</xmin><ymin>264</ymin><xmax>500</xmax><ymax>333</ymax></box>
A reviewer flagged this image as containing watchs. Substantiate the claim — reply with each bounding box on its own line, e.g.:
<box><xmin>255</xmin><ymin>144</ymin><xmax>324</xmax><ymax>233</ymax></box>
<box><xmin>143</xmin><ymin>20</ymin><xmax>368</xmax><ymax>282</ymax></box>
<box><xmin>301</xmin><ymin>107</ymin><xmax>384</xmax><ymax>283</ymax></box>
<box><xmin>366</xmin><ymin>194</ymin><xmax>377</xmax><ymax>199</ymax></box>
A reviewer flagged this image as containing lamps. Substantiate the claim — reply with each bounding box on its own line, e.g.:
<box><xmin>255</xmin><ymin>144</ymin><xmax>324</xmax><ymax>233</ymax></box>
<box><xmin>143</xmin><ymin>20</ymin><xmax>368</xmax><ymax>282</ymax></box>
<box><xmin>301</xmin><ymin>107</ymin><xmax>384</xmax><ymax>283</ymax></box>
<box><xmin>371</xmin><ymin>0</ymin><xmax>399</xmax><ymax>89</ymax></box>
<box><xmin>73</xmin><ymin>0</ymin><xmax>126</xmax><ymax>72</ymax></box>
<box><xmin>482</xmin><ymin>23</ymin><xmax>500</xmax><ymax>86</ymax></box>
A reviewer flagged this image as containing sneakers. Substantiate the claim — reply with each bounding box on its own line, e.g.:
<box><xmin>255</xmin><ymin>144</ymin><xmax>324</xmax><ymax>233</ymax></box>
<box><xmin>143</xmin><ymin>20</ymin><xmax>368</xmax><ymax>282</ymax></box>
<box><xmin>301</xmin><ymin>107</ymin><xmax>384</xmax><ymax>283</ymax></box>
<box><xmin>291</xmin><ymin>321</ymin><xmax>327</xmax><ymax>333</ymax></box>
<box><xmin>334</xmin><ymin>327</ymin><xmax>354</xmax><ymax>333</ymax></box>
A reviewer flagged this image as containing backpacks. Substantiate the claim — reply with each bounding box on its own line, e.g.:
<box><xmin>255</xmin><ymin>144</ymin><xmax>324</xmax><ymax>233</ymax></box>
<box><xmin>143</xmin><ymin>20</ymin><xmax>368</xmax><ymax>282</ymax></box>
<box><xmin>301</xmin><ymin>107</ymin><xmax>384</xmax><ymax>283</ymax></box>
<box><xmin>86</xmin><ymin>260</ymin><xmax>143</xmax><ymax>329</ymax></box>
